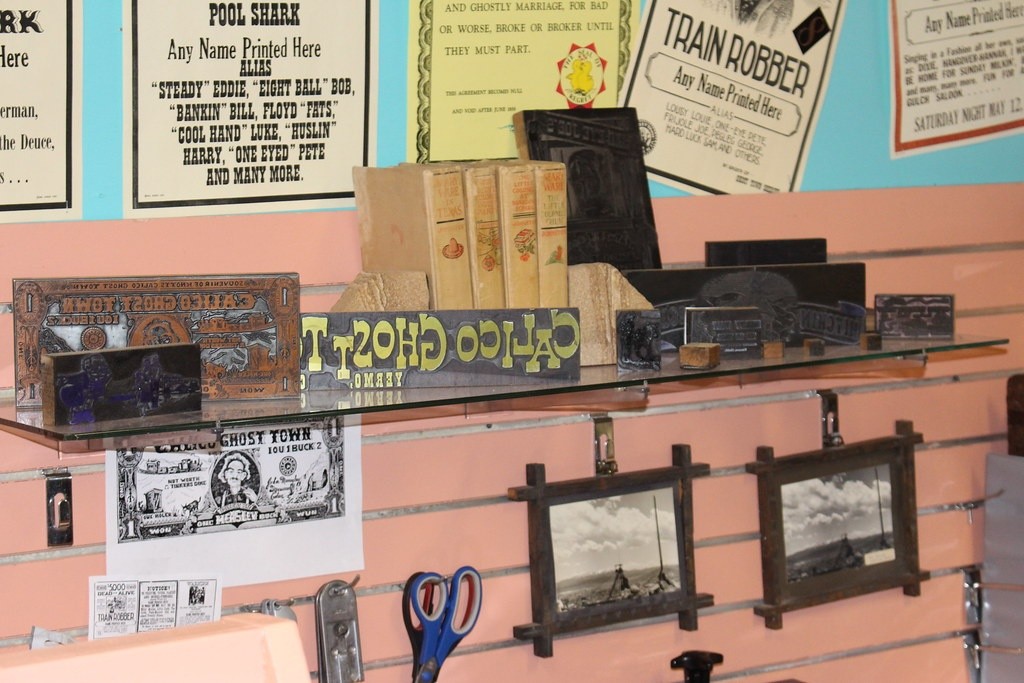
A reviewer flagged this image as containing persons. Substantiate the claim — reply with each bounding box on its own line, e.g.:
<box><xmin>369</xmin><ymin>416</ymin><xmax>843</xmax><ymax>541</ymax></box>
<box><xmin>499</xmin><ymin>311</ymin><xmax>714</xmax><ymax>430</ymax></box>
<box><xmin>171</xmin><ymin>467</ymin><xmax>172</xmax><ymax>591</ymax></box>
<box><xmin>619</xmin><ymin>574</ymin><xmax>629</xmax><ymax>590</ymax></box>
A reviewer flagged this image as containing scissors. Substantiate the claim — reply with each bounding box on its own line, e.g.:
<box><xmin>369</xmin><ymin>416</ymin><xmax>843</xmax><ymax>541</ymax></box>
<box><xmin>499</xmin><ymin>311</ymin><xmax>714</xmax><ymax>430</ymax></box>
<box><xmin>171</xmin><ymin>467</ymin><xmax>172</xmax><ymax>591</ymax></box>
<box><xmin>401</xmin><ymin>564</ymin><xmax>483</xmax><ymax>683</ymax></box>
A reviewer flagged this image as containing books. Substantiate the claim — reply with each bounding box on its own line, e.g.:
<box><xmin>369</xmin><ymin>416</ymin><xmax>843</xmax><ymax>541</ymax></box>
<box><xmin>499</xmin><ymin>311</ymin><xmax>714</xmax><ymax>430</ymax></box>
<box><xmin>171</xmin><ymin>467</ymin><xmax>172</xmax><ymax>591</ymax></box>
<box><xmin>353</xmin><ymin>159</ymin><xmax>569</xmax><ymax>311</ymax></box>
<box><xmin>513</xmin><ymin>107</ymin><xmax>663</xmax><ymax>270</ymax></box>
